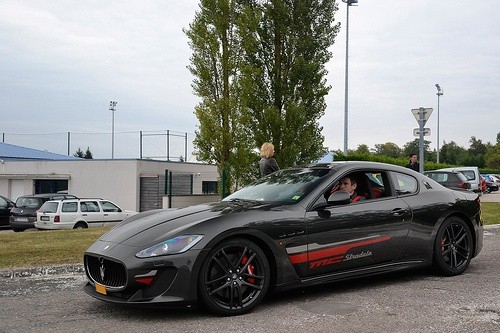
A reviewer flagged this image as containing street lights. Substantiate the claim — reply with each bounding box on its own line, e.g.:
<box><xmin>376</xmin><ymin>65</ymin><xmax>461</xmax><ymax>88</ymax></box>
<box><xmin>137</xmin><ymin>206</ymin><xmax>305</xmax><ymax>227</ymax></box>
<box><xmin>435</xmin><ymin>83</ymin><xmax>443</xmax><ymax>164</ymax></box>
<box><xmin>341</xmin><ymin>0</ymin><xmax>358</xmax><ymax>156</ymax></box>
<box><xmin>109</xmin><ymin>100</ymin><xmax>118</xmax><ymax>159</ymax></box>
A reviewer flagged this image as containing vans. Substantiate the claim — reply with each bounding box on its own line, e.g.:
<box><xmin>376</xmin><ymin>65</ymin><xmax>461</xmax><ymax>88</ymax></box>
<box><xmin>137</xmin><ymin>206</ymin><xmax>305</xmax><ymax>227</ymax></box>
<box><xmin>8</xmin><ymin>193</ymin><xmax>79</xmax><ymax>232</ymax></box>
<box><xmin>434</xmin><ymin>166</ymin><xmax>480</xmax><ymax>193</ymax></box>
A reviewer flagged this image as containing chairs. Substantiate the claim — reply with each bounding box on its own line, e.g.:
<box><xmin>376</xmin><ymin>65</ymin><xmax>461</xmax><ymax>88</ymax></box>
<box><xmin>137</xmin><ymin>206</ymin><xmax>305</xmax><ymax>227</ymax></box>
<box><xmin>23</xmin><ymin>199</ymin><xmax>31</xmax><ymax>206</ymax></box>
<box><xmin>351</xmin><ymin>172</ymin><xmax>374</xmax><ymax>200</ymax></box>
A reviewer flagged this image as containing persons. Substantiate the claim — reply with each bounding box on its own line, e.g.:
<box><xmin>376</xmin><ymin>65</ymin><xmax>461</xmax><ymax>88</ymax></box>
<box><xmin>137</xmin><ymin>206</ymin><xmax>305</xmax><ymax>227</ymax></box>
<box><xmin>339</xmin><ymin>175</ymin><xmax>363</xmax><ymax>203</ymax></box>
<box><xmin>260</xmin><ymin>143</ymin><xmax>281</xmax><ymax>200</ymax></box>
<box><xmin>408</xmin><ymin>153</ymin><xmax>420</xmax><ymax>172</ymax></box>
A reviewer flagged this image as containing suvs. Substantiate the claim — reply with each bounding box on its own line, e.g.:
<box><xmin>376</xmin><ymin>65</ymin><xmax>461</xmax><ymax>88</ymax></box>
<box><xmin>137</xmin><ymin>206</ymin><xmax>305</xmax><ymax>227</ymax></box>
<box><xmin>423</xmin><ymin>169</ymin><xmax>471</xmax><ymax>192</ymax></box>
<box><xmin>33</xmin><ymin>194</ymin><xmax>140</xmax><ymax>230</ymax></box>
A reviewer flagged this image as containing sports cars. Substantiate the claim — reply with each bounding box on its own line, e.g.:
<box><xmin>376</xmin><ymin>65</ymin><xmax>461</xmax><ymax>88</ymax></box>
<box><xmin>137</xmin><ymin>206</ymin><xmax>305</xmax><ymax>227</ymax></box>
<box><xmin>81</xmin><ymin>160</ymin><xmax>485</xmax><ymax>317</ymax></box>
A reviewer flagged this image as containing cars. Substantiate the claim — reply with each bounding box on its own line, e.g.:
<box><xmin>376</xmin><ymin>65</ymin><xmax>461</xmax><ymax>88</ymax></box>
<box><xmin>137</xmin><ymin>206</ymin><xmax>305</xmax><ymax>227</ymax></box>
<box><xmin>479</xmin><ymin>173</ymin><xmax>500</xmax><ymax>194</ymax></box>
<box><xmin>0</xmin><ymin>195</ymin><xmax>16</xmax><ymax>230</ymax></box>
